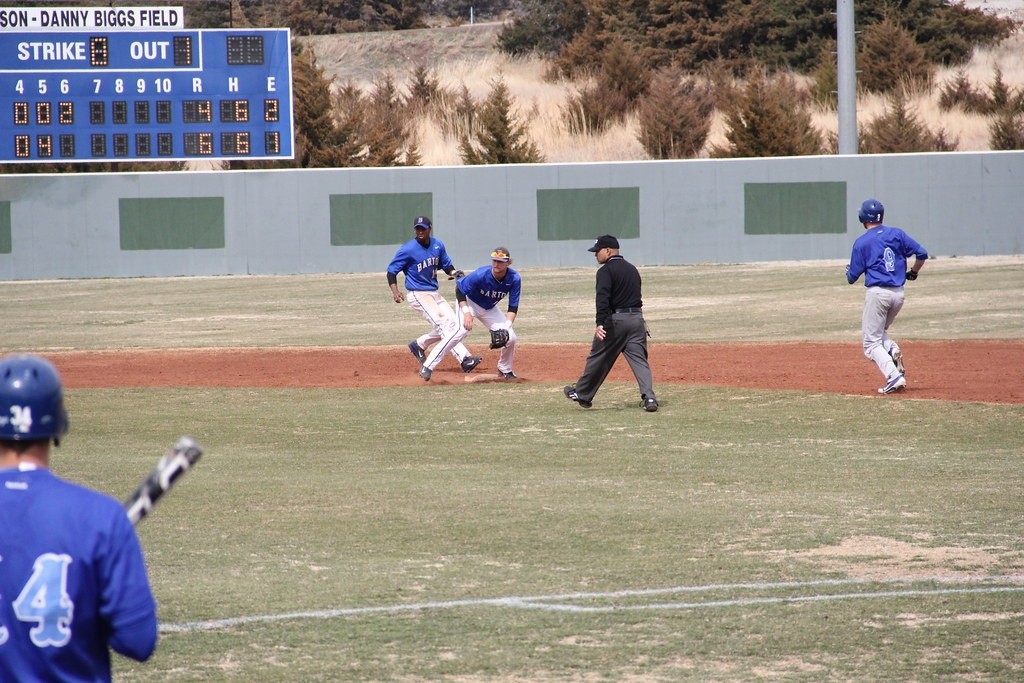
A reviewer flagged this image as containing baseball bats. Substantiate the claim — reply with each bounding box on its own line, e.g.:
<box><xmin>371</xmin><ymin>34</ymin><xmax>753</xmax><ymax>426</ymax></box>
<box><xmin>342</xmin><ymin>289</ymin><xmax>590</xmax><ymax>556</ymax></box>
<box><xmin>123</xmin><ymin>435</ymin><xmax>204</xmax><ymax>528</ymax></box>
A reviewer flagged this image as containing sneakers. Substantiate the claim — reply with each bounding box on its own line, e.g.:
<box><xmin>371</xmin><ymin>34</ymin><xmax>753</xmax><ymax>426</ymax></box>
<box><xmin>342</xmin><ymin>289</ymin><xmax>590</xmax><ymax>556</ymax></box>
<box><xmin>461</xmin><ymin>355</ymin><xmax>482</xmax><ymax>372</ymax></box>
<box><xmin>640</xmin><ymin>399</ymin><xmax>657</xmax><ymax>411</ymax></box>
<box><xmin>564</xmin><ymin>385</ymin><xmax>592</xmax><ymax>408</ymax></box>
<box><xmin>498</xmin><ymin>370</ymin><xmax>516</xmax><ymax>379</ymax></box>
<box><xmin>409</xmin><ymin>340</ymin><xmax>426</xmax><ymax>362</ymax></box>
<box><xmin>419</xmin><ymin>366</ymin><xmax>432</xmax><ymax>382</ymax></box>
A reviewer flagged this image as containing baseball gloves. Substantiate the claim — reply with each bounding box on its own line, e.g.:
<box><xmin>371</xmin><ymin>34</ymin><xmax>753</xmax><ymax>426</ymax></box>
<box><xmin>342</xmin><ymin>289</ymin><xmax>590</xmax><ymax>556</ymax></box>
<box><xmin>448</xmin><ymin>270</ymin><xmax>465</xmax><ymax>281</ymax></box>
<box><xmin>489</xmin><ymin>329</ymin><xmax>509</xmax><ymax>350</ymax></box>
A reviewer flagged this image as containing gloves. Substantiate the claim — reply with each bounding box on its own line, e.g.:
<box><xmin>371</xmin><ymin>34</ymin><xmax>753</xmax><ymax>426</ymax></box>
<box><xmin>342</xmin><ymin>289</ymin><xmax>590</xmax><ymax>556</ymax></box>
<box><xmin>906</xmin><ymin>267</ymin><xmax>918</xmax><ymax>280</ymax></box>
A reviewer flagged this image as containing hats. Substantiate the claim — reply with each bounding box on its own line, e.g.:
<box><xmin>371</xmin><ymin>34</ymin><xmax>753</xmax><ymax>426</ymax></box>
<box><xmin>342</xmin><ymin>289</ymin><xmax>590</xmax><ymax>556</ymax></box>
<box><xmin>588</xmin><ymin>233</ymin><xmax>620</xmax><ymax>252</ymax></box>
<box><xmin>490</xmin><ymin>247</ymin><xmax>510</xmax><ymax>262</ymax></box>
<box><xmin>414</xmin><ymin>216</ymin><xmax>432</xmax><ymax>229</ymax></box>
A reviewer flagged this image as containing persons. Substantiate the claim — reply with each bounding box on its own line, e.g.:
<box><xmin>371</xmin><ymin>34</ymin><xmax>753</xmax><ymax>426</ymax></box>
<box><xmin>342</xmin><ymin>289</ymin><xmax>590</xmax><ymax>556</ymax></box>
<box><xmin>845</xmin><ymin>198</ymin><xmax>929</xmax><ymax>394</ymax></box>
<box><xmin>564</xmin><ymin>234</ymin><xmax>658</xmax><ymax>412</ymax></box>
<box><xmin>420</xmin><ymin>247</ymin><xmax>521</xmax><ymax>381</ymax></box>
<box><xmin>387</xmin><ymin>217</ymin><xmax>482</xmax><ymax>373</ymax></box>
<box><xmin>0</xmin><ymin>355</ymin><xmax>156</xmax><ymax>683</ymax></box>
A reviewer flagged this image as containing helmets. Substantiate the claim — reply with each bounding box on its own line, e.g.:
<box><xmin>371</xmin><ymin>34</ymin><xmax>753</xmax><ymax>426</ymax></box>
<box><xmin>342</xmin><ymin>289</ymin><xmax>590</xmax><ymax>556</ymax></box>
<box><xmin>0</xmin><ymin>355</ymin><xmax>68</xmax><ymax>441</ymax></box>
<box><xmin>859</xmin><ymin>199</ymin><xmax>884</xmax><ymax>223</ymax></box>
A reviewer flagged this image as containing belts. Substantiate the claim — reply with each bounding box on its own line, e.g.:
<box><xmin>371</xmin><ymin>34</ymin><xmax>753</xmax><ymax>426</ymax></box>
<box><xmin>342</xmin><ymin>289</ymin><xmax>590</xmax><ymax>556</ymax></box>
<box><xmin>609</xmin><ymin>307</ymin><xmax>642</xmax><ymax>314</ymax></box>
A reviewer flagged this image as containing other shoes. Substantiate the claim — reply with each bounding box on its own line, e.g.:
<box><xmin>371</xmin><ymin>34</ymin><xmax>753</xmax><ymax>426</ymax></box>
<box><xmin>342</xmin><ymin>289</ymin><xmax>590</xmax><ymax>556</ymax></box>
<box><xmin>878</xmin><ymin>375</ymin><xmax>906</xmax><ymax>394</ymax></box>
<box><xmin>888</xmin><ymin>347</ymin><xmax>905</xmax><ymax>376</ymax></box>
